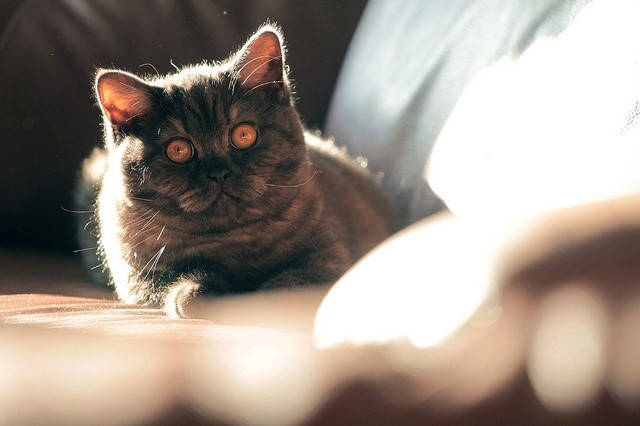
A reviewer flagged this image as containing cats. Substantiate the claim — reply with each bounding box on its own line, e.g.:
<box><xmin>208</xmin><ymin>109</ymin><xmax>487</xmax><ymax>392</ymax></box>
<box><xmin>60</xmin><ymin>17</ymin><xmax>404</xmax><ymax>320</ymax></box>
